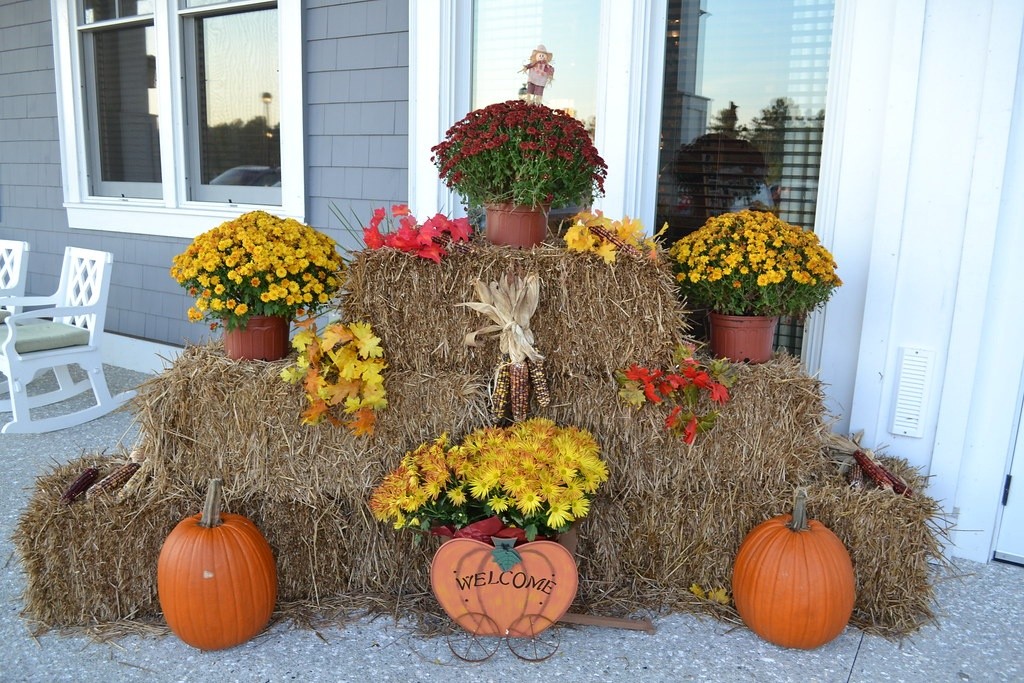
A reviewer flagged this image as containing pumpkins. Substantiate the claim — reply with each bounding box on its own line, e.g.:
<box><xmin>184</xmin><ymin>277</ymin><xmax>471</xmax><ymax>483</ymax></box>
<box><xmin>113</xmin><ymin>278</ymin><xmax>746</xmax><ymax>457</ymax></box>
<box><xmin>733</xmin><ymin>486</ymin><xmax>854</xmax><ymax>648</ymax></box>
<box><xmin>157</xmin><ymin>477</ymin><xmax>277</xmax><ymax>650</ymax></box>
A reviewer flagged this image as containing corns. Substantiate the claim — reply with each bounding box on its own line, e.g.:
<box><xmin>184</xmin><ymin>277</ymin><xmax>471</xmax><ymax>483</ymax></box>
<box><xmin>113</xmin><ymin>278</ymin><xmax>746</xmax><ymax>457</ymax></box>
<box><xmin>85</xmin><ymin>462</ymin><xmax>141</xmax><ymax>499</ymax></box>
<box><xmin>59</xmin><ymin>467</ymin><xmax>99</xmax><ymax>505</ymax></box>
<box><xmin>117</xmin><ymin>465</ymin><xmax>152</xmax><ymax>499</ymax></box>
<box><xmin>847</xmin><ymin>450</ymin><xmax>915</xmax><ymax>497</ymax></box>
<box><xmin>490</xmin><ymin>354</ymin><xmax>550</xmax><ymax>424</ymax></box>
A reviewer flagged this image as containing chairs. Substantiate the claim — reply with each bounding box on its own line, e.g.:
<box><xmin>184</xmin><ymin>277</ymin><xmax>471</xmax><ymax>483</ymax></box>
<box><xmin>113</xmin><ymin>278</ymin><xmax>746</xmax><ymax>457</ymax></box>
<box><xmin>0</xmin><ymin>245</ymin><xmax>135</xmax><ymax>433</ymax></box>
<box><xmin>0</xmin><ymin>239</ymin><xmax>29</xmax><ymax>340</ymax></box>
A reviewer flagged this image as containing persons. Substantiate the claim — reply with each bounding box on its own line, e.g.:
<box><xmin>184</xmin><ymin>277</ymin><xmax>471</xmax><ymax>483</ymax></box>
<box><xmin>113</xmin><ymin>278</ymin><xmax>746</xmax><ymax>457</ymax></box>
<box><xmin>517</xmin><ymin>44</ymin><xmax>555</xmax><ymax>107</ymax></box>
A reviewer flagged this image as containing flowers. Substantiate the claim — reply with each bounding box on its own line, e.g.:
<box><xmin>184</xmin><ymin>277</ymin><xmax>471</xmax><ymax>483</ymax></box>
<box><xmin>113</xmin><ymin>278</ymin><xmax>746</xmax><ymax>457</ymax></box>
<box><xmin>362</xmin><ymin>201</ymin><xmax>477</xmax><ymax>265</ymax></box>
<box><xmin>562</xmin><ymin>207</ymin><xmax>672</xmax><ymax>266</ymax></box>
<box><xmin>290</xmin><ymin>318</ymin><xmax>395</xmax><ymax>440</ymax></box>
<box><xmin>609</xmin><ymin>350</ymin><xmax>732</xmax><ymax>445</ymax></box>
<box><xmin>173</xmin><ymin>210</ymin><xmax>346</xmax><ymax>332</ymax></box>
<box><xmin>430</xmin><ymin>99</ymin><xmax>608</xmax><ymax>217</ymax></box>
<box><xmin>674</xmin><ymin>215</ymin><xmax>839</xmax><ymax>324</ymax></box>
<box><xmin>666</xmin><ymin>134</ymin><xmax>771</xmax><ymax>225</ymax></box>
<box><xmin>370</xmin><ymin>413</ymin><xmax>611</xmax><ymax>553</ymax></box>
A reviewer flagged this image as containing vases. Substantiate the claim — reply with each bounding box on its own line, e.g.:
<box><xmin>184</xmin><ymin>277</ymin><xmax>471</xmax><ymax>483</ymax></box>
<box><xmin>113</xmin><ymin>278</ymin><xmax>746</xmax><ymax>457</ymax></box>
<box><xmin>222</xmin><ymin>315</ymin><xmax>289</xmax><ymax>361</ymax></box>
<box><xmin>713</xmin><ymin>311</ymin><xmax>776</xmax><ymax>364</ymax></box>
<box><xmin>482</xmin><ymin>204</ymin><xmax>551</xmax><ymax>249</ymax></box>
